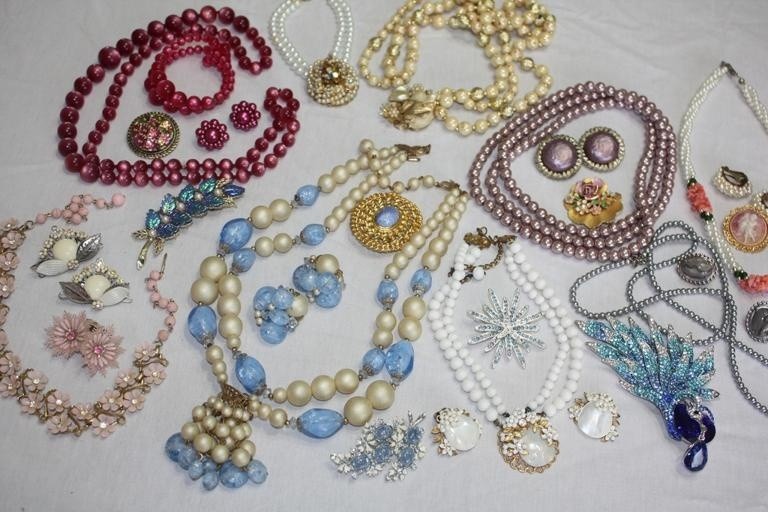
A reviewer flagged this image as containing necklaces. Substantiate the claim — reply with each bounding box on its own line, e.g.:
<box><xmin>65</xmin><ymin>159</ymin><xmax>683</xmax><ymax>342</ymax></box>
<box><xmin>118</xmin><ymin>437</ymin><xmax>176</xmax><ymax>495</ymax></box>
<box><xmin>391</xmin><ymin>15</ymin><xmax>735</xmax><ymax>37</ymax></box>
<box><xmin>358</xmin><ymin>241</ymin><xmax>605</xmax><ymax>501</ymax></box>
<box><xmin>1</xmin><ymin>0</ymin><xmax>767</xmax><ymax>492</ymax></box>
